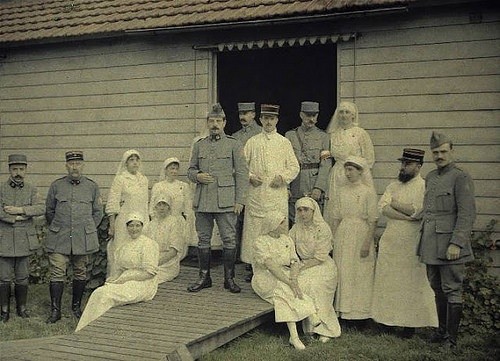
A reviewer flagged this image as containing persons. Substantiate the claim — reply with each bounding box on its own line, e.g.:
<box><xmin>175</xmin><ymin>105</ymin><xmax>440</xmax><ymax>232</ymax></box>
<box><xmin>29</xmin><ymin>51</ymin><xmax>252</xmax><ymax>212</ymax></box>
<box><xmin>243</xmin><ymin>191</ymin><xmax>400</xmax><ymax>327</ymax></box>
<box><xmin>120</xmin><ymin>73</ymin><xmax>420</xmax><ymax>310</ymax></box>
<box><xmin>250</xmin><ymin>211</ymin><xmax>316</xmax><ymax>349</ymax></box>
<box><xmin>185</xmin><ymin>103</ymin><xmax>247</xmax><ymax>292</ymax></box>
<box><xmin>326</xmin><ymin>155</ymin><xmax>379</xmax><ymax>333</ymax></box>
<box><xmin>187</xmin><ymin>127</ymin><xmax>224</xmax><ymax>263</ymax></box>
<box><xmin>321</xmin><ymin>102</ymin><xmax>376</xmax><ymax>235</ymax></box>
<box><xmin>142</xmin><ymin>194</ymin><xmax>184</xmax><ymax>283</ymax></box>
<box><xmin>232</xmin><ymin>103</ymin><xmax>262</xmax><ymax>145</ymax></box>
<box><xmin>240</xmin><ymin>104</ymin><xmax>300</xmax><ymax>283</ymax></box>
<box><xmin>148</xmin><ymin>157</ymin><xmax>193</xmax><ymax>263</ymax></box>
<box><xmin>0</xmin><ymin>154</ymin><xmax>46</xmax><ymax>322</ymax></box>
<box><xmin>288</xmin><ymin>197</ymin><xmax>341</xmax><ymax>342</ymax></box>
<box><xmin>46</xmin><ymin>150</ymin><xmax>106</xmax><ymax>324</ymax></box>
<box><xmin>285</xmin><ymin>102</ymin><xmax>331</xmax><ymax>229</ymax></box>
<box><xmin>105</xmin><ymin>149</ymin><xmax>150</xmax><ymax>280</ymax></box>
<box><xmin>416</xmin><ymin>129</ymin><xmax>477</xmax><ymax>352</ymax></box>
<box><xmin>370</xmin><ymin>148</ymin><xmax>440</xmax><ymax>338</ymax></box>
<box><xmin>75</xmin><ymin>213</ymin><xmax>160</xmax><ymax>333</ymax></box>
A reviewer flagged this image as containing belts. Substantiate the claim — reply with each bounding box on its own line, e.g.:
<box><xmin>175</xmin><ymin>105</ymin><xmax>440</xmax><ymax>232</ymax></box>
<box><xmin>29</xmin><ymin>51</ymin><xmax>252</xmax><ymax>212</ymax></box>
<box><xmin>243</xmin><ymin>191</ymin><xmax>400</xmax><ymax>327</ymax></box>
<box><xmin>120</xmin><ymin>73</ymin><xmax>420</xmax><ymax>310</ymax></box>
<box><xmin>300</xmin><ymin>163</ymin><xmax>319</xmax><ymax>169</ymax></box>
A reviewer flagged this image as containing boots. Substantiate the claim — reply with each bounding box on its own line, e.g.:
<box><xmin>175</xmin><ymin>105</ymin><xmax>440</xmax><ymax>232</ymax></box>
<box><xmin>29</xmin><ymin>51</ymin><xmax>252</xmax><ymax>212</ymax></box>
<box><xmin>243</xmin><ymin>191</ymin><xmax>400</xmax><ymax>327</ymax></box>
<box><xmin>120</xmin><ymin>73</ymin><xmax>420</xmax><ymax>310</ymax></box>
<box><xmin>223</xmin><ymin>248</ymin><xmax>241</xmax><ymax>293</ymax></box>
<box><xmin>72</xmin><ymin>280</ymin><xmax>85</xmax><ymax>319</ymax></box>
<box><xmin>46</xmin><ymin>281</ymin><xmax>63</xmax><ymax>323</ymax></box>
<box><xmin>187</xmin><ymin>247</ymin><xmax>212</xmax><ymax>292</ymax></box>
<box><xmin>0</xmin><ymin>286</ymin><xmax>11</xmax><ymax>323</ymax></box>
<box><xmin>427</xmin><ymin>303</ymin><xmax>447</xmax><ymax>343</ymax></box>
<box><xmin>440</xmin><ymin>303</ymin><xmax>462</xmax><ymax>348</ymax></box>
<box><xmin>287</xmin><ymin>323</ymin><xmax>305</xmax><ymax>349</ymax></box>
<box><xmin>14</xmin><ymin>286</ymin><xmax>30</xmax><ymax>318</ymax></box>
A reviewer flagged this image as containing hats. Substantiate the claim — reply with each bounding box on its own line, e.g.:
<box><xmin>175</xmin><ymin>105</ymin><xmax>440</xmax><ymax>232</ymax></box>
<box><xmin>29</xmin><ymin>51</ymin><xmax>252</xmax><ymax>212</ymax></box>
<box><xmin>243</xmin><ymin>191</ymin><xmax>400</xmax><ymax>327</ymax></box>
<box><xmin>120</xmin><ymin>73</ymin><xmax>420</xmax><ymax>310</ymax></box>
<box><xmin>7</xmin><ymin>154</ymin><xmax>27</xmax><ymax>165</ymax></box>
<box><xmin>207</xmin><ymin>102</ymin><xmax>226</xmax><ymax>118</ymax></box>
<box><xmin>238</xmin><ymin>103</ymin><xmax>255</xmax><ymax>112</ymax></box>
<box><xmin>260</xmin><ymin>104</ymin><xmax>279</xmax><ymax>115</ymax></box>
<box><xmin>301</xmin><ymin>102</ymin><xmax>319</xmax><ymax>112</ymax></box>
<box><xmin>65</xmin><ymin>151</ymin><xmax>83</xmax><ymax>161</ymax></box>
<box><xmin>397</xmin><ymin>148</ymin><xmax>425</xmax><ymax>163</ymax></box>
<box><xmin>430</xmin><ymin>131</ymin><xmax>451</xmax><ymax>149</ymax></box>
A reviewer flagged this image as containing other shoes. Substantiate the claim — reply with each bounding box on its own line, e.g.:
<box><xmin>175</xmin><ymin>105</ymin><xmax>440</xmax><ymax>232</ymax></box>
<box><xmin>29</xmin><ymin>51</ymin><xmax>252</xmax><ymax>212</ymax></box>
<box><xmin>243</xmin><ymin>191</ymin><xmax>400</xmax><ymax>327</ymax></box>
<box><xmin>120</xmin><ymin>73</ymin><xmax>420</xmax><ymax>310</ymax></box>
<box><xmin>403</xmin><ymin>327</ymin><xmax>415</xmax><ymax>337</ymax></box>
<box><xmin>378</xmin><ymin>325</ymin><xmax>394</xmax><ymax>333</ymax></box>
<box><xmin>319</xmin><ymin>336</ymin><xmax>330</xmax><ymax>343</ymax></box>
<box><xmin>245</xmin><ymin>274</ymin><xmax>253</xmax><ymax>282</ymax></box>
<box><xmin>247</xmin><ymin>264</ymin><xmax>252</xmax><ymax>271</ymax></box>
